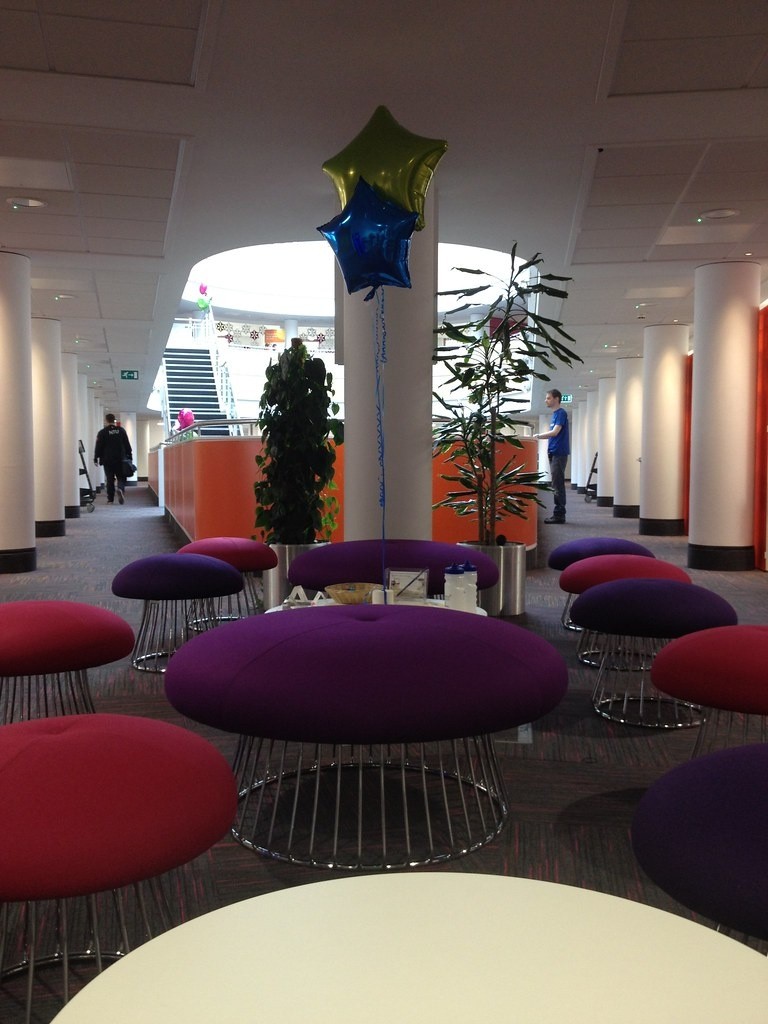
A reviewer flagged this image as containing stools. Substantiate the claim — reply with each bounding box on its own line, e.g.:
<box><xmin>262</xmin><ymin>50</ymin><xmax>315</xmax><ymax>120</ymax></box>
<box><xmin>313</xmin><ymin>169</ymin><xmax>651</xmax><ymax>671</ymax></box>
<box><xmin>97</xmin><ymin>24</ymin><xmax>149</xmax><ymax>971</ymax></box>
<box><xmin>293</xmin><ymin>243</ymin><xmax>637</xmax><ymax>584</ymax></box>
<box><xmin>559</xmin><ymin>556</ymin><xmax>692</xmax><ymax>673</ymax></box>
<box><xmin>178</xmin><ymin>538</ymin><xmax>278</xmax><ymax>633</ymax></box>
<box><xmin>112</xmin><ymin>554</ymin><xmax>245</xmax><ymax>674</ymax></box>
<box><xmin>651</xmin><ymin>624</ymin><xmax>768</xmax><ymax>760</ymax></box>
<box><xmin>0</xmin><ymin>714</ymin><xmax>238</xmax><ymax>1024</ymax></box>
<box><xmin>48</xmin><ymin>871</ymin><xmax>768</xmax><ymax>1024</ymax></box>
<box><xmin>570</xmin><ymin>578</ymin><xmax>738</xmax><ymax>729</ymax></box>
<box><xmin>630</xmin><ymin>740</ymin><xmax>768</xmax><ymax>955</ymax></box>
<box><xmin>0</xmin><ymin>600</ymin><xmax>135</xmax><ymax>724</ymax></box>
<box><xmin>287</xmin><ymin>538</ymin><xmax>500</xmax><ymax>593</ymax></box>
<box><xmin>163</xmin><ymin>604</ymin><xmax>570</xmax><ymax>873</ymax></box>
<box><xmin>548</xmin><ymin>537</ymin><xmax>655</xmax><ymax>633</ymax></box>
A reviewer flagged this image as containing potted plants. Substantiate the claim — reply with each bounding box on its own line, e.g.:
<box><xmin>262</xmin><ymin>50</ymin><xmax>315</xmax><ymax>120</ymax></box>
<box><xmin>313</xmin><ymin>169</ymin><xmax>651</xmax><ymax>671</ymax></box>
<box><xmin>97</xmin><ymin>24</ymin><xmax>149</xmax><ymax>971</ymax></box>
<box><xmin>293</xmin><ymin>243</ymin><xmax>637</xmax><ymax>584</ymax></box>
<box><xmin>426</xmin><ymin>241</ymin><xmax>586</xmax><ymax>618</ymax></box>
<box><xmin>249</xmin><ymin>336</ymin><xmax>345</xmax><ymax>612</ymax></box>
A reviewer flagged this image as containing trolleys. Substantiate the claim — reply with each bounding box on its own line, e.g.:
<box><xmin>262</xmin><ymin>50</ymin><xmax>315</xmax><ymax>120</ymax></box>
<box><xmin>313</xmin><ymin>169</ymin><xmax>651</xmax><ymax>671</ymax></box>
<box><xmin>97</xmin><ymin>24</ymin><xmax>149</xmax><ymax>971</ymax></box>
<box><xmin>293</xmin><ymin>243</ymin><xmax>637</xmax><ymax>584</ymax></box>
<box><xmin>78</xmin><ymin>438</ymin><xmax>97</xmax><ymax>514</ymax></box>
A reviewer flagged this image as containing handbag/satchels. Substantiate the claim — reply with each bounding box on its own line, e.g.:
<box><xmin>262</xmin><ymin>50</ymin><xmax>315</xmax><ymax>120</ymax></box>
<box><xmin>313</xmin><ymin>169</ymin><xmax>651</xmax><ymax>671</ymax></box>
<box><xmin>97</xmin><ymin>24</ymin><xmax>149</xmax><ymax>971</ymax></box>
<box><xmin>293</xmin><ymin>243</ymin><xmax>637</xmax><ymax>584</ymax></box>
<box><xmin>122</xmin><ymin>459</ymin><xmax>136</xmax><ymax>478</ymax></box>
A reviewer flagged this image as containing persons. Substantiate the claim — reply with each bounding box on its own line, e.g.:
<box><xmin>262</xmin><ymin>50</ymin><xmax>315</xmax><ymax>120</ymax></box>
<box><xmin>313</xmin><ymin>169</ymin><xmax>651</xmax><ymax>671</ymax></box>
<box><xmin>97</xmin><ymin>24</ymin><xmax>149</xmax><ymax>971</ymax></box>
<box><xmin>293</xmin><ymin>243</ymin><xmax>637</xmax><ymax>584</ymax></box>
<box><xmin>534</xmin><ymin>389</ymin><xmax>572</xmax><ymax>523</ymax></box>
<box><xmin>94</xmin><ymin>414</ymin><xmax>132</xmax><ymax>505</ymax></box>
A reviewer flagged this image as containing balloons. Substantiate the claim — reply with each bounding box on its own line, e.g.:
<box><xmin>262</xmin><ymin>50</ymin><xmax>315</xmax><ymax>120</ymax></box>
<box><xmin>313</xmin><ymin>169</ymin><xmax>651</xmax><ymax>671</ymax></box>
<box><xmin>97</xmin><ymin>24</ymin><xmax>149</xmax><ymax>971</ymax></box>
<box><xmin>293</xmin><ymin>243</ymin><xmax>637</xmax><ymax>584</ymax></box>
<box><xmin>197</xmin><ymin>283</ymin><xmax>210</xmax><ymax>310</ymax></box>
<box><xmin>177</xmin><ymin>409</ymin><xmax>195</xmax><ymax>432</ymax></box>
<box><xmin>314</xmin><ymin>105</ymin><xmax>448</xmax><ymax>299</ymax></box>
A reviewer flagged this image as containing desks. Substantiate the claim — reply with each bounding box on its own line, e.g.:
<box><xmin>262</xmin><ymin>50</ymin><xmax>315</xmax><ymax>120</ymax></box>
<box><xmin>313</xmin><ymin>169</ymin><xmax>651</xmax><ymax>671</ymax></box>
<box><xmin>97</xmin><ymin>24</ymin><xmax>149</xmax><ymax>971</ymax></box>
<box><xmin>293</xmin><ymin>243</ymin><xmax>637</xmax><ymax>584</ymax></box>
<box><xmin>265</xmin><ymin>598</ymin><xmax>488</xmax><ymax>618</ymax></box>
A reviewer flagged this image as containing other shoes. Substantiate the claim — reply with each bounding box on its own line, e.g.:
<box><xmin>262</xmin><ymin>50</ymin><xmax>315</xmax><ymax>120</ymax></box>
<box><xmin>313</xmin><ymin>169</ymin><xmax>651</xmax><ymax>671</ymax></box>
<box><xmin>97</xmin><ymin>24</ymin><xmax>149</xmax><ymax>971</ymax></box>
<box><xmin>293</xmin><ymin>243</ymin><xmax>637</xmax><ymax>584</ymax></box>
<box><xmin>108</xmin><ymin>500</ymin><xmax>113</xmax><ymax>505</ymax></box>
<box><xmin>117</xmin><ymin>489</ymin><xmax>125</xmax><ymax>505</ymax></box>
<box><xmin>544</xmin><ymin>515</ymin><xmax>565</xmax><ymax>524</ymax></box>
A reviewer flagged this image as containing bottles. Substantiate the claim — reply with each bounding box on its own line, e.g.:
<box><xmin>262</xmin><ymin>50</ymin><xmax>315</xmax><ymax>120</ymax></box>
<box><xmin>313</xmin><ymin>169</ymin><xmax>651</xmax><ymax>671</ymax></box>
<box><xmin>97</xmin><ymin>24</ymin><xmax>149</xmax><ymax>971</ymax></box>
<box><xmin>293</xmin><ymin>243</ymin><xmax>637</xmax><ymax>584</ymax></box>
<box><xmin>459</xmin><ymin>560</ymin><xmax>477</xmax><ymax>614</ymax></box>
<box><xmin>444</xmin><ymin>562</ymin><xmax>465</xmax><ymax>611</ymax></box>
